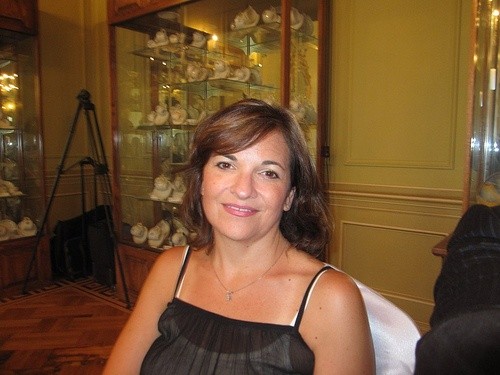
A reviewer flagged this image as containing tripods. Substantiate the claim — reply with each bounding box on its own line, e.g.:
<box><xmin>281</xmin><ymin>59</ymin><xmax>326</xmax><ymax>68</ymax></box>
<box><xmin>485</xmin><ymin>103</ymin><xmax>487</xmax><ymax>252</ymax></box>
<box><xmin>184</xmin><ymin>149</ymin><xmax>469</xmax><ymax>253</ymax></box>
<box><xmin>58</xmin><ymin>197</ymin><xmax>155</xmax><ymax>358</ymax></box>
<box><xmin>23</xmin><ymin>100</ymin><xmax>132</xmax><ymax>307</ymax></box>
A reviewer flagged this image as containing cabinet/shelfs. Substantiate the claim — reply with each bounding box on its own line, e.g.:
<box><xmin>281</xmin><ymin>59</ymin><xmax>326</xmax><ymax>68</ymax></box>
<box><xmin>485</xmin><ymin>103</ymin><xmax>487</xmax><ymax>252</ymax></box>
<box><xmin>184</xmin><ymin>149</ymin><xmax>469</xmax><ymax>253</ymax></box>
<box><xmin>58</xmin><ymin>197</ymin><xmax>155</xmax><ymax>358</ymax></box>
<box><xmin>125</xmin><ymin>19</ymin><xmax>320</xmax><ymax>256</ymax></box>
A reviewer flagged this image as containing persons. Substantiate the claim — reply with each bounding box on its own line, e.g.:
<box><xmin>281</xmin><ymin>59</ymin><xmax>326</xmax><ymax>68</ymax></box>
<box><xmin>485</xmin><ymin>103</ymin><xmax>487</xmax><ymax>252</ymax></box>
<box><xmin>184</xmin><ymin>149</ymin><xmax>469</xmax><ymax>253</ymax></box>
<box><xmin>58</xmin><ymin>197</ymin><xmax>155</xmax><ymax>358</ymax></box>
<box><xmin>101</xmin><ymin>98</ymin><xmax>375</xmax><ymax>375</ymax></box>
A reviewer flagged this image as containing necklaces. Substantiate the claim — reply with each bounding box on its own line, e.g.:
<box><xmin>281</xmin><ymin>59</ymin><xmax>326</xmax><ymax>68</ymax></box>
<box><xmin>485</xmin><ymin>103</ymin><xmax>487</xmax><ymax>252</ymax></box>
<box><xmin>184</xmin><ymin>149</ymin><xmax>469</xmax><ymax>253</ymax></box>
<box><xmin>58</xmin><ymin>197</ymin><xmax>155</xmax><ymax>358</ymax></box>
<box><xmin>208</xmin><ymin>239</ymin><xmax>288</xmax><ymax>302</ymax></box>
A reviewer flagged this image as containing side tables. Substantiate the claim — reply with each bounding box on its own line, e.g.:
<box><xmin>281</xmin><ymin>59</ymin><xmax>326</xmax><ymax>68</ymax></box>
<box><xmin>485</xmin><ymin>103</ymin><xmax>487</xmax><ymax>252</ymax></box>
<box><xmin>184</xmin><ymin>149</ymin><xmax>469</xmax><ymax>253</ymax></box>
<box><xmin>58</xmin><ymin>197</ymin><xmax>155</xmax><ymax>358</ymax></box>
<box><xmin>1</xmin><ymin>124</ymin><xmax>39</xmax><ymax>243</ymax></box>
